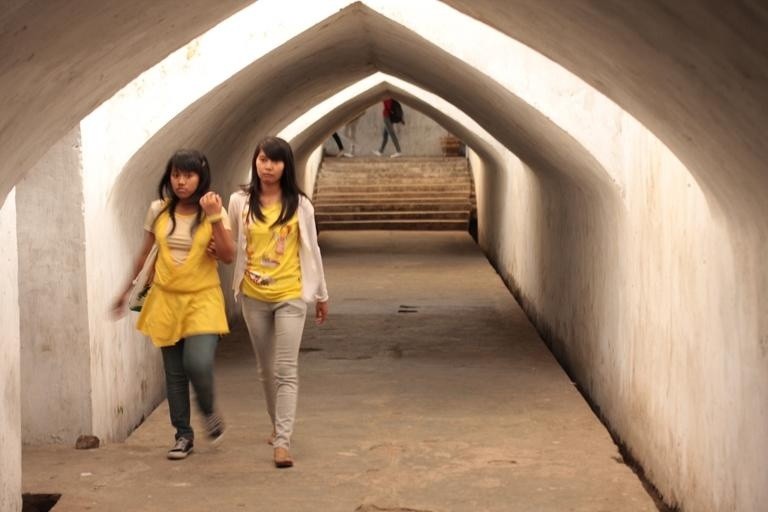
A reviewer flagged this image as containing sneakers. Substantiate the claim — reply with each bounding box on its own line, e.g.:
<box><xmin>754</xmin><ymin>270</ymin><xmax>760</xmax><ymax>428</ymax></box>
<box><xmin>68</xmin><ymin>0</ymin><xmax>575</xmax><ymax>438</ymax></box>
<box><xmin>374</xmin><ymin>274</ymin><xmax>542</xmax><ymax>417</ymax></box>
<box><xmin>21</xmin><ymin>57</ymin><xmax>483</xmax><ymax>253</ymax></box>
<box><xmin>390</xmin><ymin>153</ymin><xmax>403</xmax><ymax>158</ymax></box>
<box><xmin>374</xmin><ymin>150</ymin><xmax>382</xmax><ymax>158</ymax></box>
<box><xmin>274</xmin><ymin>446</ymin><xmax>292</xmax><ymax>468</ymax></box>
<box><xmin>268</xmin><ymin>431</ymin><xmax>276</xmax><ymax>445</ymax></box>
<box><xmin>346</xmin><ymin>153</ymin><xmax>355</xmax><ymax>158</ymax></box>
<box><xmin>337</xmin><ymin>151</ymin><xmax>345</xmax><ymax>158</ymax></box>
<box><xmin>167</xmin><ymin>438</ymin><xmax>193</xmax><ymax>459</ymax></box>
<box><xmin>206</xmin><ymin>414</ymin><xmax>225</xmax><ymax>446</ymax></box>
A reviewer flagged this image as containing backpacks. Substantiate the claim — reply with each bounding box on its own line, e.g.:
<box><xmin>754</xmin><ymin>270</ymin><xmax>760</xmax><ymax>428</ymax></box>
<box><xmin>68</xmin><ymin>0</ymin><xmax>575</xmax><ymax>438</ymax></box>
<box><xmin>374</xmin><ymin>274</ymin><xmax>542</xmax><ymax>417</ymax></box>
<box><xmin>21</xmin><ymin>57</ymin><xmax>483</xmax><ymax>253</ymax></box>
<box><xmin>390</xmin><ymin>100</ymin><xmax>402</xmax><ymax>123</ymax></box>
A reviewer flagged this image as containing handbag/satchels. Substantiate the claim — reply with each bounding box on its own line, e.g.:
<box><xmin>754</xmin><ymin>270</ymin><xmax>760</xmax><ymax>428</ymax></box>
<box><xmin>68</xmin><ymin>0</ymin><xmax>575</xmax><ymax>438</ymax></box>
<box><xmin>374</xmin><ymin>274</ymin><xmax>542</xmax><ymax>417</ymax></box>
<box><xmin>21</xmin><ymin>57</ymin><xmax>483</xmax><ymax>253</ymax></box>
<box><xmin>128</xmin><ymin>245</ymin><xmax>160</xmax><ymax>310</ymax></box>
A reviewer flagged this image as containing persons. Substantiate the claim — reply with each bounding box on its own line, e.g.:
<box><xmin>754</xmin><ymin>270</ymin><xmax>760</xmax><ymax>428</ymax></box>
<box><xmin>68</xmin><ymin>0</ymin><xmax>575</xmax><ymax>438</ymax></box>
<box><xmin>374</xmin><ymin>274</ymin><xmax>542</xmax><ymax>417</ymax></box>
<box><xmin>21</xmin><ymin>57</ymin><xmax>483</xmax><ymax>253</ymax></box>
<box><xmin>371</xmin><ymin>97</ymin><xmax>403</xmax><ymax>158</ymax></box>
<box><xmin>341</xmin><ymin>118</ymin><xmax>364</xmax><ymax>158</ymax></box>
<box><xmin>107</xmin><ymin>149</ymin><xmax>236</xmax><ymax>460</ymax></box>
<box><xmin>203</xmin><ymin>136</ymin><xmax>328</xmax><ymax>470</ymax></box>
<box><xmin>331</xmin><ymin>131</ymin><xmax>346</xmax><ymax>159</ymax></box>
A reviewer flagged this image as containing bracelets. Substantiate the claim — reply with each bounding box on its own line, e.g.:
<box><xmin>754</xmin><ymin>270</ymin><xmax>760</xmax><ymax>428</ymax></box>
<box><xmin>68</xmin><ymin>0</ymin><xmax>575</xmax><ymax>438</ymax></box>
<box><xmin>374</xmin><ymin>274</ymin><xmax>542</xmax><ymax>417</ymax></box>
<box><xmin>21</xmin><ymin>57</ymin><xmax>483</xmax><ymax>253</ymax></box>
<box><xmin>206</xmin><ymin>215</ymin><xmax>225</xmax><ymax>225</ymax></box>
